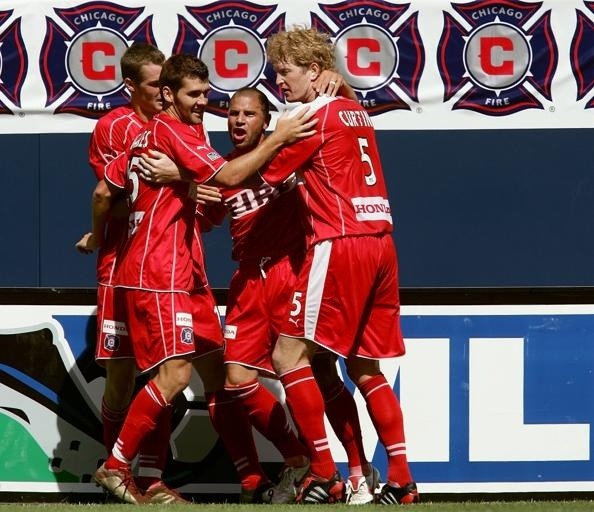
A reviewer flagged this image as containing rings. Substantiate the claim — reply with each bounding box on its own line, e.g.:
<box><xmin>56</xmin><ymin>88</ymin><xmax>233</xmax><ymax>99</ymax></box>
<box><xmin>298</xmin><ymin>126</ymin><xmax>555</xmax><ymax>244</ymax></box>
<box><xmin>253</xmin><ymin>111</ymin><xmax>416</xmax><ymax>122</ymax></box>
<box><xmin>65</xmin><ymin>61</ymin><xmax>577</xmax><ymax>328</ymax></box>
<box><xmin>143</xmin><ymin>170</ymin><xmax>149</xmax><ymax>177</ymax></box>
<box><xmin>328</xmin><ymin>81</ymin><xmax>335</xmax><ymax>85</ymax></box>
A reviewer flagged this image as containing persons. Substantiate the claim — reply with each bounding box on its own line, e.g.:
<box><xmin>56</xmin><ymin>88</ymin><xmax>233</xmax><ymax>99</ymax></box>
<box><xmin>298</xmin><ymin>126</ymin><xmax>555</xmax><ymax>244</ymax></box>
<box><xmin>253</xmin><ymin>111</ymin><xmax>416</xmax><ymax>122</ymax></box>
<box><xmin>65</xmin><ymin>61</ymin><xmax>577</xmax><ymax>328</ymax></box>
<box><xmin>193</xmin><ymin>69</ymin><xmax>379</xmax><ymax>506</ymax></box>
<box><xmin>91</xmin><ymin>41</ymin><xmax>282</xmax><ymax>502</ymax></box>
<box><xmin>130</xmin><ymin>27</ymin><xmax>420</xmax><ymax>507</ymax></box>
<box><xmin>72</xmin><ymin>52</ymin><xmax>321</xmax><ymax>506</ymax></box>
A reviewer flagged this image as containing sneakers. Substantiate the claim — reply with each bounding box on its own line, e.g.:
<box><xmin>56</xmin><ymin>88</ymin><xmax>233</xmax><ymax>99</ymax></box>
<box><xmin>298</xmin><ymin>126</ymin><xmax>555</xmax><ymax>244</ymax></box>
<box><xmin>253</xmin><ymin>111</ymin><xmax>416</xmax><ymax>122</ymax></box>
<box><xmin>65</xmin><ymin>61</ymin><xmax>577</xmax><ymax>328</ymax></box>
<box><xmin>242</xmin><ymin>458</ymin><xmax>419</xmax><ymax>504</ymax></box>
<box><xmin>136</xmin><ymin>477</ymin><xmax>193</xmax><ymax>505</ymax></box>
<box><xmin>90</xmin><ymin>463</ymin><xmax>146</xmax><ymax>504</ymax></box>
